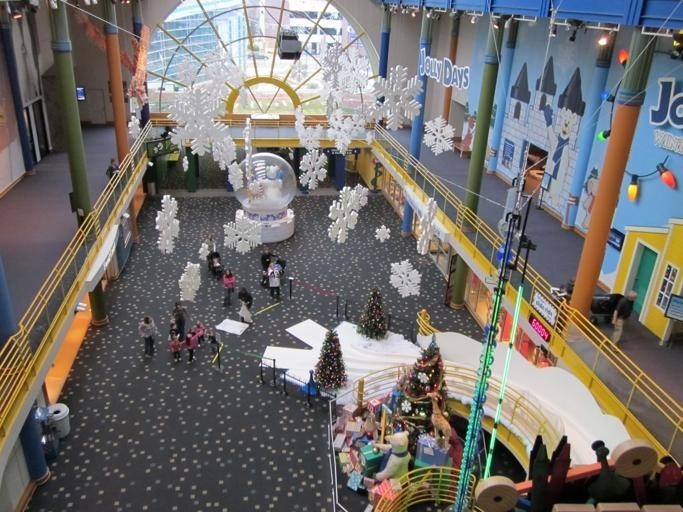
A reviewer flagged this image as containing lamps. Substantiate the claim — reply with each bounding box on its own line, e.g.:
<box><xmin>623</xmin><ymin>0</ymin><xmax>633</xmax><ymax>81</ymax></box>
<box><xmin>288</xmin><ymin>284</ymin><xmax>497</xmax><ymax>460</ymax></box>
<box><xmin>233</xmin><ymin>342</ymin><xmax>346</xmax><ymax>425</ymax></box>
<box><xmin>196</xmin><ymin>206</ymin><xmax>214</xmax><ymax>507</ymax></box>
<box><xmin>471</xmin><ymin>15</ymin><xmax>477</xmax><ymax>24</ymax></box>
<box><xmin>569</xmin><ymin>30</ymin><xmax>576</xmax><ymax>42</ymax></box>
<box><xmin>379</xmin><ymin>4</ymin><xmax>432</xmax><ymax>18</ymax></box>
<box><xmin>493</xmin><ymin>20</ymin><xmax>499</xmax><ymax>29</ymax></box>
<box><xmin>504</xmin><ymin>17</ymin><xmax>513</xmax><ymax>29</ymax></box>
<box><xmin>549</xmin><ymin>25</ymin><xmax>557</xmax><ymax>37</ymax></box>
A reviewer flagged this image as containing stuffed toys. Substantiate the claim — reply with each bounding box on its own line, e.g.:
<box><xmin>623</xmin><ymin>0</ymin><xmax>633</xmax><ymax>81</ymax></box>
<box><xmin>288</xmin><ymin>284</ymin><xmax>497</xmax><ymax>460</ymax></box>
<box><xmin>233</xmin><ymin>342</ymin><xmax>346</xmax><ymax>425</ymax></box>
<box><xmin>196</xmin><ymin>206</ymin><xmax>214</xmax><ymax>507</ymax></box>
<box><xmin>428</xmin><ymin>392</ymin><xmax>451</xmax><ymax>447</ymax></box>
<box><xmin>376</xmin><ymin>431</ymin><xmax>412</xmax><ymax>483</ymax></box>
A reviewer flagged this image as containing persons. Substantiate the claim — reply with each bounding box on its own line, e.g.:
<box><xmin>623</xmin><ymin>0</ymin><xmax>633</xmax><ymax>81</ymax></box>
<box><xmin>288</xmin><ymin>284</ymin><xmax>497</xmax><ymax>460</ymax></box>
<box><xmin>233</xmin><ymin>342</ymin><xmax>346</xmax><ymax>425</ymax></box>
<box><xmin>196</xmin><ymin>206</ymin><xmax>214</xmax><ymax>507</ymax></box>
<box><xmin>260</xmin><ymin>247</ymin><xmax>286</xmax><ymax>300</ymax></box>
<box><xmin>206</xmin><ymin>249</ymin><xmax>223</xmax><ymax>280</ymax></box>
<box><xmin>106</xmin><ymin>159</ymin><xmax>121</xmax><ymax>190</ymax></box>
<box><xmin>555</xmin><ymin>278</ymin><xmax>575</xmax><ymax>310</ymax></box>
<box><xmin>222</xmin><ymin>268</ymin><xmax>236</xmax><ymax>307</ymax></box>
<box><xmin>610</xmin><ymin>291</ymin><xmax>637</xmax><ymax>353</ymax></box>
<box><xmin>136</xmin><ymin>316</ymin><xmax>156</xmax><ymax>358</ymax></box>
<box><xmin>238</xmin><ymin>287</ymin><xmax>253</xmax><ymax>322</ymax></box>
<box><xmin>168</xmin><ymin>302</ymin><xmax>206</xmax><ymax>365</ymax></box>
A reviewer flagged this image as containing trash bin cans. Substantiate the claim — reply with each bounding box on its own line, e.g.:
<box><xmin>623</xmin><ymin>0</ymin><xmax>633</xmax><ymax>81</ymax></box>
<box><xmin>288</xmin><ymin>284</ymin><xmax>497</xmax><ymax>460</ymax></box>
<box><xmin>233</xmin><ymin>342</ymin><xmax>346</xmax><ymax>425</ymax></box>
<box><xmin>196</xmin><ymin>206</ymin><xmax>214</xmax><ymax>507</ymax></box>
<box><xmin>46</xmin><ymin>403</ymin><xmax>71</xmax><ymax>439</ymax></box>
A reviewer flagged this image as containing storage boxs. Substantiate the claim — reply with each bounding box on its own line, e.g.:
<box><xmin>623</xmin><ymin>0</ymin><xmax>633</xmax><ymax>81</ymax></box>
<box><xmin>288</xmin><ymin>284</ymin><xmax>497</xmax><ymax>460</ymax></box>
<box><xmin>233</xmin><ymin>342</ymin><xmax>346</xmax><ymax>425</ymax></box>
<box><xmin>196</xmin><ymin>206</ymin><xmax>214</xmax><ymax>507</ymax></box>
<box><xmin>333</xmin><ymin>393</ymin><xmax>452</xmax><ymax>511</ymax></box>
